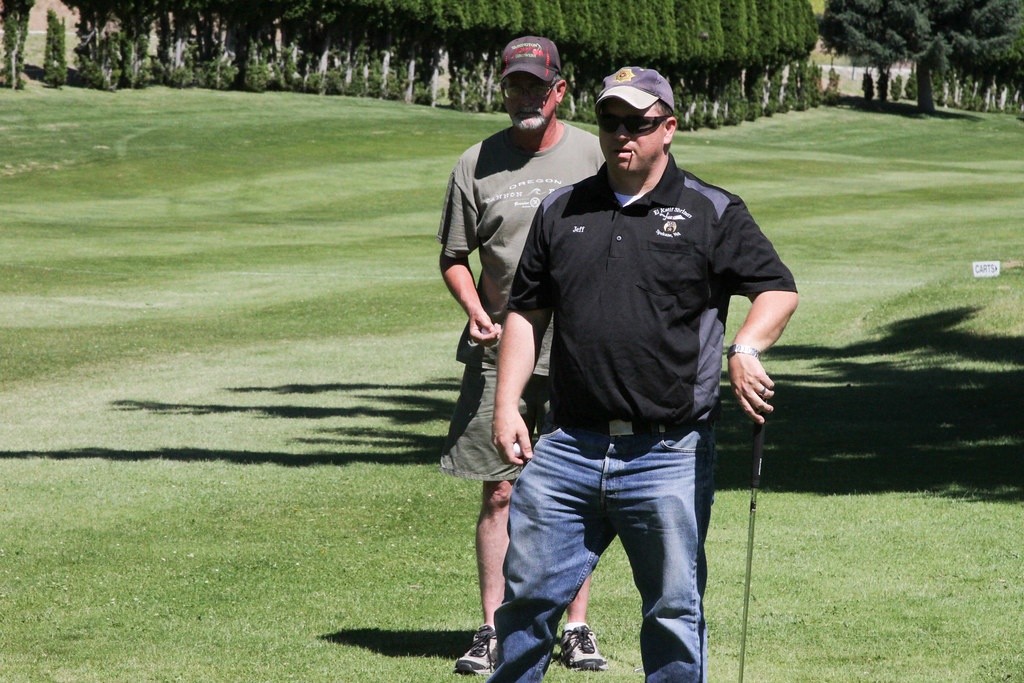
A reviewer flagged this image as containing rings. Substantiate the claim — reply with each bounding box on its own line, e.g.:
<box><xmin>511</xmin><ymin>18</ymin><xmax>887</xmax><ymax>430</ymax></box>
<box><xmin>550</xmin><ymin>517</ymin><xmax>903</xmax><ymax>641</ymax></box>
<box><xmin>757</xmin><ymin>386</ymin><xmax>766</xmax><ymax>396</ymax></box>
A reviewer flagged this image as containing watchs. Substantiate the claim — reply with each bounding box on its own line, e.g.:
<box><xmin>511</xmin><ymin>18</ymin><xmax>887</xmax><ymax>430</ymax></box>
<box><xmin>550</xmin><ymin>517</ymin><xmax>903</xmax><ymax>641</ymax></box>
<box><xmin>726</xmin><ymin>344</ymin><xmax>760</xmax><ymax>359</ymax></box>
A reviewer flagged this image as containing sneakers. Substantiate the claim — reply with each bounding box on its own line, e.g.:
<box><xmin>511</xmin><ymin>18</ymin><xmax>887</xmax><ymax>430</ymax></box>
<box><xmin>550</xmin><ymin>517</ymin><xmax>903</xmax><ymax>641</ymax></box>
<box><xmin>454</xmin><ymin>624</ymin><xmax>498</xmax><ymax>675</ymax></box>
<box><xmin>550</xmin><ymin>624</ymin><xmax>609</xmax><ymax>670</ymax></box>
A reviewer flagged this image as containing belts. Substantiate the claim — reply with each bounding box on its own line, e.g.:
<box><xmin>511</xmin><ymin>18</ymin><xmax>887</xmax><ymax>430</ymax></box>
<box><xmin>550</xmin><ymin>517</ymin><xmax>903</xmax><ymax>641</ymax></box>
<box><xmin>555</xmin><ymin>411</ymin><xmax>674</xmax><ymax>438</ymax></box>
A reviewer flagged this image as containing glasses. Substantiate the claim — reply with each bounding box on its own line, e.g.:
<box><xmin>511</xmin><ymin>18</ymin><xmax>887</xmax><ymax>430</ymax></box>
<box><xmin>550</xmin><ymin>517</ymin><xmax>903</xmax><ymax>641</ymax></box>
<box><xmin>597</xmin><ymin>111</ymin><xmax>671</xmax><ymax>134</ymax></box>
<box><xmin>503</xmin><ymin>79</ymin><xmax>560</xmax><ymax>99</ymax></box>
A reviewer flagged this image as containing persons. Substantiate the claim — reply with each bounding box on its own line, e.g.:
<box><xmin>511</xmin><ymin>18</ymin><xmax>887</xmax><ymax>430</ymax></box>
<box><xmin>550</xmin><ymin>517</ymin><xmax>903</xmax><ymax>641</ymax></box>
<box><xmin>437</xmin><ymin>37</ymin><xmax>800</xmax><ymax>683</ymax></box>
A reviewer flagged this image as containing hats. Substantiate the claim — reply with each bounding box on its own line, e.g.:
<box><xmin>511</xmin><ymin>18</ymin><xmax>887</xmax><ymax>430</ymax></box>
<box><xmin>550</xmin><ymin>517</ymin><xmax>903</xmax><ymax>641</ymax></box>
<box><xmin>595</xmin><ymin>66</ymin><xmax>675</xmax><ymax>117</ymax></box>
<box><xmin>499</xmin><ymin>36</ymin><xmax>562</xmax><ymax>81</ymax></box>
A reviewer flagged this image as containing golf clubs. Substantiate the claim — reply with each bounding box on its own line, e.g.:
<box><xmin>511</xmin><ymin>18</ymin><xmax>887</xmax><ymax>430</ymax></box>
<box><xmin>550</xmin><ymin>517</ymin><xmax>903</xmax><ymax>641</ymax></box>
<box><xmin>740</xmin><ymin>404</ymin><xmax>768</xmax><ymax>683</ymax></box>
